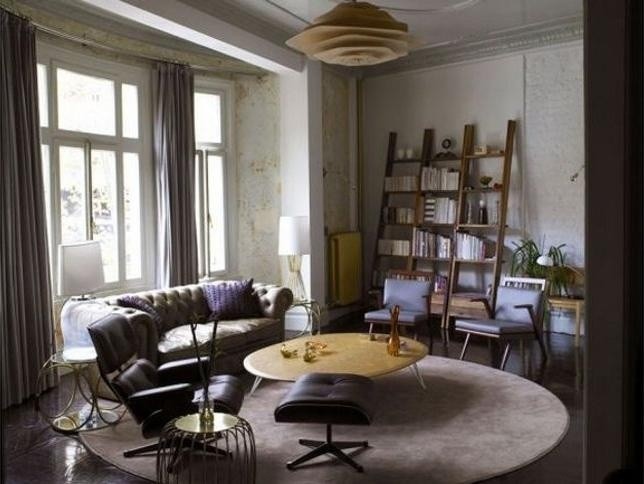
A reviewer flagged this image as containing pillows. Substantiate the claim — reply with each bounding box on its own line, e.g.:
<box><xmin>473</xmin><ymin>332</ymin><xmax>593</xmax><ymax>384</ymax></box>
<box><xmin>198</xmin><ymin>276</ymin><xmax>264</xmax><ymax>321</ymax></box>
<box><xmin>115</xmin><ymin>293</ymin><xmax>167</xmax><ymax>337</ymax></box>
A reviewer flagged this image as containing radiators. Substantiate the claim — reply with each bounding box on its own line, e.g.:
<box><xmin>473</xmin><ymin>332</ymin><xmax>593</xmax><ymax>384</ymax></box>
<box><xmin>324</xmin><ymin>228</ymin><xmax>364</xmax><ymax>311</ymax></box>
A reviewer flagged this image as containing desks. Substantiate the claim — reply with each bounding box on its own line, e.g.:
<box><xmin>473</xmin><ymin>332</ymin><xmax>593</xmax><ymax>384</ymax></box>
<box><xmin>286</xmin><ymin>299</ymin><xmax>323</xmax><ymax>341</ymax></box>
<box><xmin>35</xmin><ymin>353</ymin><xmax>132</xmax><ymax>435</ymax></box>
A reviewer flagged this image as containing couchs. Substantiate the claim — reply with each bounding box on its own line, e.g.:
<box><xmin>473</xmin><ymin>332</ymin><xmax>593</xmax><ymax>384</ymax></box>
<box><xmin>83</xmin><ymin>280</ymin><xmax>293</xmax><ymax>404</ymax></box>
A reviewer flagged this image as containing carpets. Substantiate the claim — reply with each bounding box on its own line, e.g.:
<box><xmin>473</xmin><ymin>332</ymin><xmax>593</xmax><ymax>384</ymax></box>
<box><xmin>80</xmin><ymin>354</ymin><xmax>571</xmax><ymax>482</ymax></box>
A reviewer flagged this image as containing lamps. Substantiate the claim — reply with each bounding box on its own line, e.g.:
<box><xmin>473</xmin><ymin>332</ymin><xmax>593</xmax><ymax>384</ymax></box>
<box><xmin>277</xmin><ymin>213</ymin><xmax>312</xmax><ymax>304</ymax></box>
<box><xmin>281</xmin><ymin>2</ymin><xmax>412</xmax><ymax>68</ymax></box>
<box><xmin>55</xmin><ymin>239</ymin><xmax>103</xmax><ymax>301</ymax></box>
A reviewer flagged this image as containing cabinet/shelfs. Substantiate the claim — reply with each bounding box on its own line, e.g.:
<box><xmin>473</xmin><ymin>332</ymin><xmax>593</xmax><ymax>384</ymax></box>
<box><xmin>367</xmin><ymin>120</ymin><xmax>514</xmax><ymax>332</ymax></box>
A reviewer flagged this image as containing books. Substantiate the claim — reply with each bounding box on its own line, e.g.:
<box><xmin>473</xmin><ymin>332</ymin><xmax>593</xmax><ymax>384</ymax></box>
<box><xmin>373</xmin><ymin>166</ymin><xmax>495</xmax><ymax>297</ymax></box>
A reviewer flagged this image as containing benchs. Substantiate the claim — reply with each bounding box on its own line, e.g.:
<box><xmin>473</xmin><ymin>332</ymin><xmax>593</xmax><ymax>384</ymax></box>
<box><xmin>521</xmin><ymin>266</ymin><xmax>586</xmax><ymax>349</ymax></box>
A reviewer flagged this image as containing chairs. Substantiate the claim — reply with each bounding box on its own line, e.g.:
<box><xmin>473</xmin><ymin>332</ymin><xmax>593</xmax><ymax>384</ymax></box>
<box><xmin>364</xmin><ymin>278</ymin><xmax>433</xmax><ymax>354</ymax></box>
<box><xmin>87</xmin><ymin>313</ymin><xmax>372</xmax><ymax>473</ymax></box>
<box><xmin>455</xmin><ymin>284</ymin><xmax>548</xmax><ymax>370</ymax></box>
